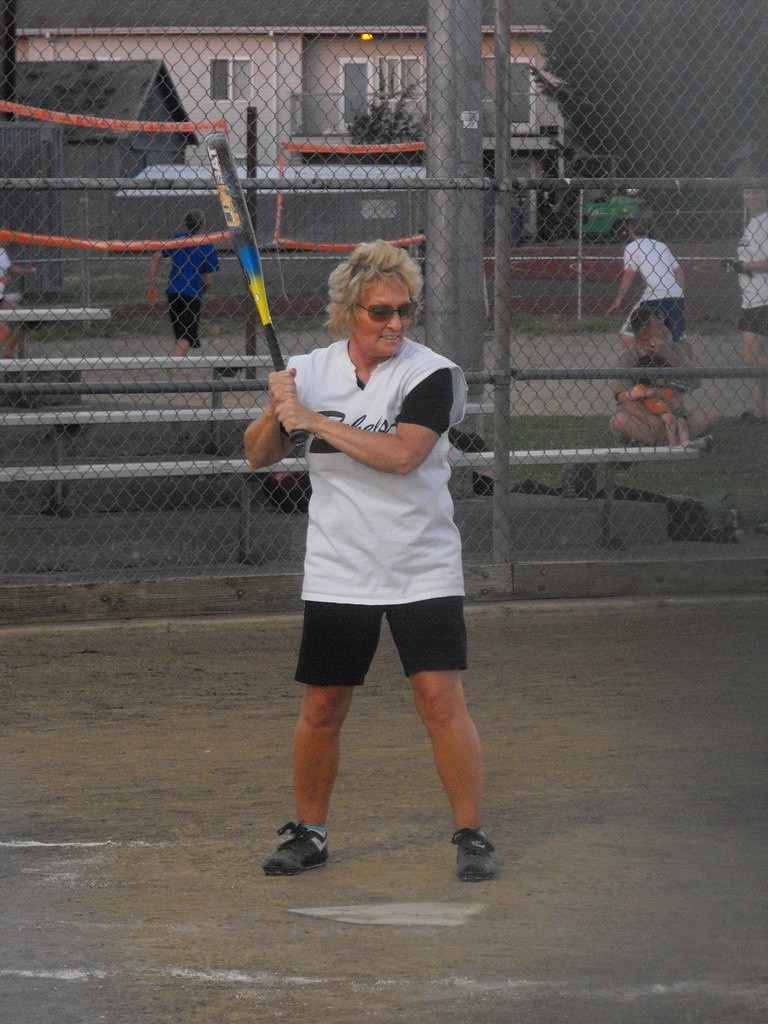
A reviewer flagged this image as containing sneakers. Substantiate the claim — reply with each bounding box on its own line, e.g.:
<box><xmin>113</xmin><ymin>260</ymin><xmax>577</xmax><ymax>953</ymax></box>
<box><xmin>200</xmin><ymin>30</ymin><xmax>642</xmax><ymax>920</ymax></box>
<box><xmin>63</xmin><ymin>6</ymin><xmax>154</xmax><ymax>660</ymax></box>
<box><xmin>264</xmin><ymin>822</ymin><xmax>329</xmax><ymax>875</ymax></box>
<box><xmin>452</xmin><ymin>828</ymin><xmax>497</xmax><ymax>882</ymax></box>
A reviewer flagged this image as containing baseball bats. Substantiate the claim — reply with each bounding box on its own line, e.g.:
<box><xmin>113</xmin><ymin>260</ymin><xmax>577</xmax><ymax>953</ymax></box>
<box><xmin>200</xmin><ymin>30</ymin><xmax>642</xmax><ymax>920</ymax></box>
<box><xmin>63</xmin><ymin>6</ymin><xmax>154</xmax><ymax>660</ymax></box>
<box><xmin>204</xmin><ymin>135</ymin><xmax>307</xmax><ymax>444</ymax></box>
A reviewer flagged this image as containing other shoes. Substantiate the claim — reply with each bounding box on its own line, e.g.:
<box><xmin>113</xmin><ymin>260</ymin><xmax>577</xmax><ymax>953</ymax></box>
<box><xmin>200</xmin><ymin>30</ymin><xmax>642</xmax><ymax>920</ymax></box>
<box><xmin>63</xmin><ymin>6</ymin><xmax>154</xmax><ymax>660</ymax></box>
<box><xmin>691</xmin><ymin>434</ymin><xmax>714</xmax><ymax>456</ymax></box>
<box><xmin>737</xmin><ymin>409</ymin><xmax>768</xmax><ymax>427</ymax></box>
<box><xmin>681</xmin><ymin>439</ymin><xmax>695</xmax><ymax>448</ymax></box>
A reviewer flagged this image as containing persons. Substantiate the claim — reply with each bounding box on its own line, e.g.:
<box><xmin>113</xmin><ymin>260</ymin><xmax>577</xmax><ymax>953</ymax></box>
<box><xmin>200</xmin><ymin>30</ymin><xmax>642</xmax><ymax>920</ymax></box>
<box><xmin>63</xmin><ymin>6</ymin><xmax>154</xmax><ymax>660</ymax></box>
<box><xmin>721</xmin><ymin>172</ymin><xmax>768</xmax><ymax>424</ymax></box>
<box><xmin>146</xmin><ymin>208</ymin><xmax>220</xmax><ymax>379</ymax></box>
<box><xmin>608</xmin><ymin>306</ymin><xmax>714</xmax><ymax>453</ymax></box>
<box><xmin>0</xmin><ymin>243</ymin><xmax>37</xmax><ymax>359</ymax></box>
<box><xmin>243</xmin><ymin>238</ymin><xmax>496</xmax><ymax>882</ymax></box>
<box><xmin>603</xmin><ymin>216</ymin><xmax>698</xmax><ymax>365</ymax></box>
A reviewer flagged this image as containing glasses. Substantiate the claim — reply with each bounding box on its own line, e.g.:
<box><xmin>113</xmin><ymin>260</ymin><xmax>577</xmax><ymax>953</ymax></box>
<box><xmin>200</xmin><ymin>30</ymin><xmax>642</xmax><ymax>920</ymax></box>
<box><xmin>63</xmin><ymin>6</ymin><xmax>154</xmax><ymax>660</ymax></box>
<box><xmin>358</xmin><ymin>298</ymin><xmax>418</xmax><ymax>322</ymax></box>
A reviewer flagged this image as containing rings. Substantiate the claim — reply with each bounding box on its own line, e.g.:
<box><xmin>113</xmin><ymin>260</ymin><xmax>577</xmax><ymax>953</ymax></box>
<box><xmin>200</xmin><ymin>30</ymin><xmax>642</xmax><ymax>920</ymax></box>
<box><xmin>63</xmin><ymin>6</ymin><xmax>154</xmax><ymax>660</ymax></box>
<box><xmin>651</xmin><ymin>343</ymin><xmax>655</xmax><ymax>348</ymax></box>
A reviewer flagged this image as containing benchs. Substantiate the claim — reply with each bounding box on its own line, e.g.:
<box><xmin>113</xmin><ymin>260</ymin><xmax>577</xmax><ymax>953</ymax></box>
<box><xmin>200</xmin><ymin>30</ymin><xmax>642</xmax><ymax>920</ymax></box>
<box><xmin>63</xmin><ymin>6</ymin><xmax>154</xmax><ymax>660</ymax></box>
<box><xmin>0</xmin><ymin>309</ymin><xmax>698</xmax><ymax>563</ymax></box>
